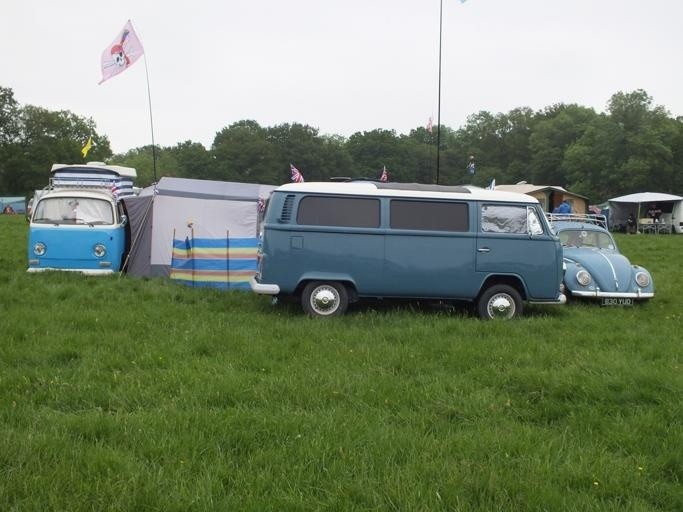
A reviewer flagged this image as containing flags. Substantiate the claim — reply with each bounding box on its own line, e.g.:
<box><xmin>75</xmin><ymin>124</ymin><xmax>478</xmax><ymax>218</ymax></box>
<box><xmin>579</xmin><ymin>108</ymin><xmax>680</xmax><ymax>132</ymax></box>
<box><xmin>81</xmin><ymin>136</ymin><xmax>92</xmax><ymax>158</ymax></box>
<box><xmin>380</xmin><ymin>166</ymin><xmax>388</xmax><ymax>181</ymax></box>
<box><xmin>427</xmin><ymin>118</ymin><xmax>433</xmax><ymax>133</ymax></box>
<box><xmin>489</xmin><ymin>178</ymin><xmax>495</xmax><ymax>190</ymax></box>
<box><xmin>289</xmin><ymin>163</ymin><xmax>304</xmax><ymax>183</ymax></box>
<box><xmin>96</xmin><ymin>20</ymin><xmax>145</xmax><ymax>86</ymax></box>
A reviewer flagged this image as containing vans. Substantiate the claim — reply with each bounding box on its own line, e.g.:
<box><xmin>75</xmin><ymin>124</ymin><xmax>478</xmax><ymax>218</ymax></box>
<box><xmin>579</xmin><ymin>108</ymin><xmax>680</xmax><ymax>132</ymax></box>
<box><xmin>26</xmin><ymin>179</ymin><xmax>130</xmax><ymax>276</ymax></box>
<box><xmin>248</xmin><ymin>180</ymin><xmax>567</xmax><ymax>319</ymax></box>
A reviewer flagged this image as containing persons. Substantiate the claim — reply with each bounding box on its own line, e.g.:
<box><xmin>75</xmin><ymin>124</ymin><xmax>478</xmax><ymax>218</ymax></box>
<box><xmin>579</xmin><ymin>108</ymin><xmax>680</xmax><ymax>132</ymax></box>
<box><xmin>626</xmin><ymin>213</ymin><xmax>641</xmax><ymax>235</ymax></box>
<box><xmin>552</xmin><ymin>199</ymin><xmax>571</xmax><ymax>213</ymax></box>
<box><xmin>647</xmin><ymin>204</ymin><xmax>662</xmax><ymax>223</ymax></box>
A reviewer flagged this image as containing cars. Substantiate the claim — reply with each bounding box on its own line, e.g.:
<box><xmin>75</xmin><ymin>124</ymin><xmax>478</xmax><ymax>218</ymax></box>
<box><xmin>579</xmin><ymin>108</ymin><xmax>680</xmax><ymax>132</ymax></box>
<box><xmin>544</xmin><ymin>208</ymin><xmax>653</xmax><ymax>306</ymax></box>
